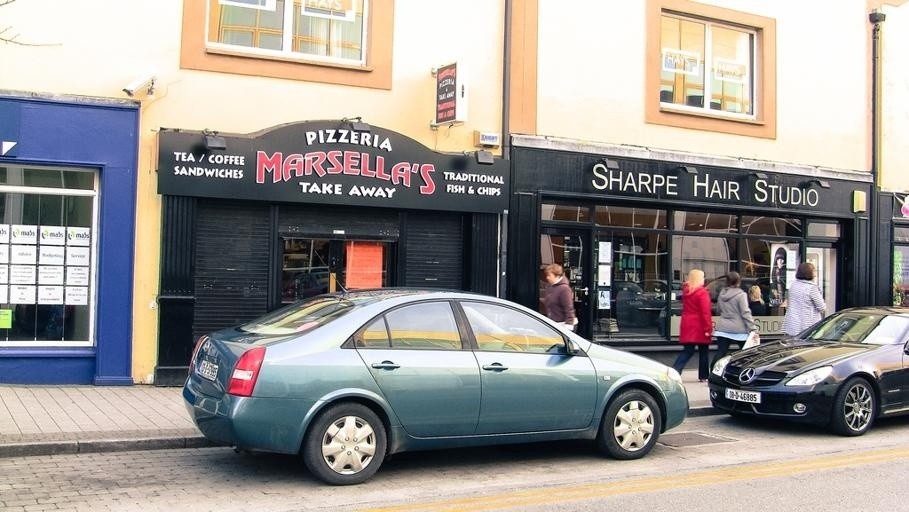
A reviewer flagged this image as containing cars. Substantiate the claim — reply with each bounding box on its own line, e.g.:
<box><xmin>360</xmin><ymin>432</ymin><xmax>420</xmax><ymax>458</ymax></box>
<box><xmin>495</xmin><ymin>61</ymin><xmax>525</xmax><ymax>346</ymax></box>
<box><xmin>615</xmin><ymin>275</ymin><xmax>770</xmax><ymax>338</ymax></box>
<box><xmin>183</xmin><ymin>288</ymin><xmax>688</xmax><ymax>486</ymax></box>
<box><xmin>709</xmin><ymin>304</ymin><xmax>908</xmax><ymax>437</ymax></box>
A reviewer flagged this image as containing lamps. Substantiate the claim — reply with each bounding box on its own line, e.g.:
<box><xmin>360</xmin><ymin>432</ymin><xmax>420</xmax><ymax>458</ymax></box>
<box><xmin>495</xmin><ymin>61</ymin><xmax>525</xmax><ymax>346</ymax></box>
<box><xmin>601</xmin><ymin>156</ymin><xmax>832</xmax><ymax>190</ymax></box>
<box><xmin>464</xmin><ymin>149</ymin><xmax>494</xmax><ymax>165</ymax></box>
<box><xmin>342</xmin><ymin>115</ymin><xmax>371</xmax><ymax>133</ymax></box>
<box><xmin>202</xmin><ymin>129</ymin><xmax>227</xmax><ymax>151</ymax></box>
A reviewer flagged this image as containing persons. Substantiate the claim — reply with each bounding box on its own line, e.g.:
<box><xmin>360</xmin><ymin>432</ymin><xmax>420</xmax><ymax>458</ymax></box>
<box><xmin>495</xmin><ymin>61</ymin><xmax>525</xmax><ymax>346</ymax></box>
<box><xmin>781</xmin><ymin>261</ymin><xmax>827</xmax><ymax>338</ymax></box>
<box><xmin>710</xmin><ymin>270</ymin><xmax>759</xmax><ymax>371</ymax></box>
<box><xmin>671</xmin><ymin>268</ymin><xmax>715</xmax><ymax>383</ymax></box>
<box><xmin>746</xmin><ymin>285</ymin><xmax>767</xmax><ymax>315</ymax></box>
<box><xmin>543</xmin><ymin>262</ymin><xmax>578</xmax><ymax>334</ymax></box>
<box><xmin>770</xmin><ymin>247</ymin><xmax>787</xmax><ymax>305</ymax></box>
